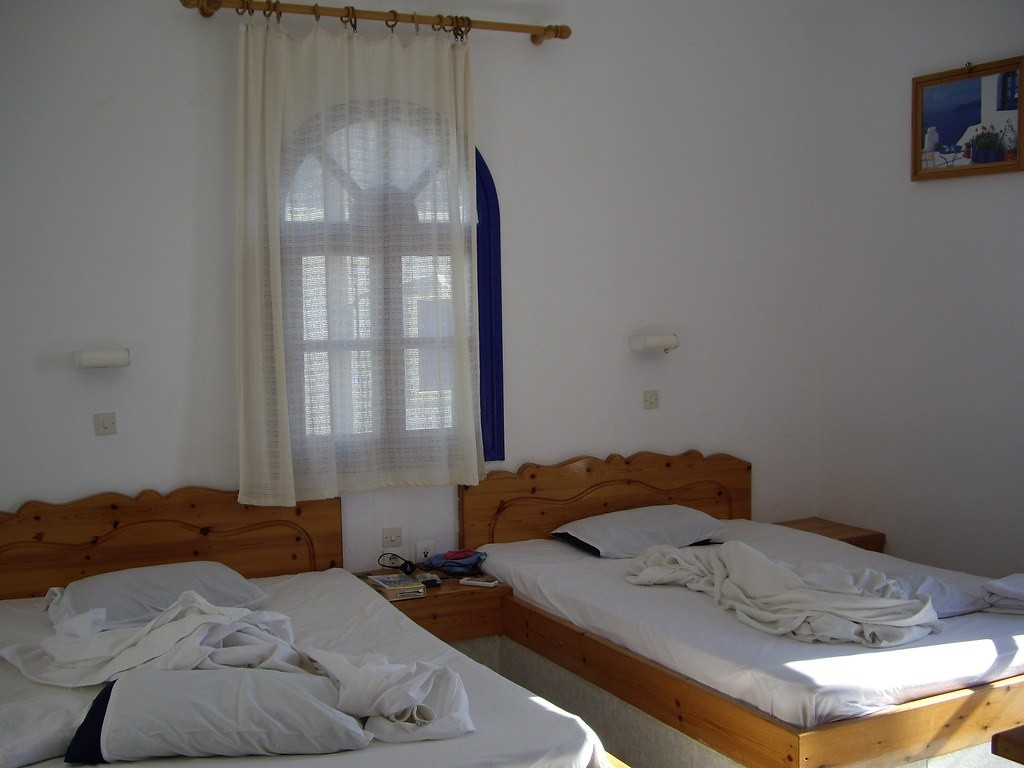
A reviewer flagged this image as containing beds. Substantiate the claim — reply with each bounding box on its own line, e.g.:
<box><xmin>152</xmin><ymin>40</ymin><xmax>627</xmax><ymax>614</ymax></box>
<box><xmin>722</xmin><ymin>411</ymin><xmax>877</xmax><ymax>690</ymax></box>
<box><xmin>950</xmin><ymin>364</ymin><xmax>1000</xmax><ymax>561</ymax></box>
<box><xmin>457</xmin><ymin>448</ymin><xmax>1024</xmax><ymax>768</ymax></box>
<box><xmin>0</xmin><ymin>487</ymin><xmax>630</xmax><ymax>768</ymax></box>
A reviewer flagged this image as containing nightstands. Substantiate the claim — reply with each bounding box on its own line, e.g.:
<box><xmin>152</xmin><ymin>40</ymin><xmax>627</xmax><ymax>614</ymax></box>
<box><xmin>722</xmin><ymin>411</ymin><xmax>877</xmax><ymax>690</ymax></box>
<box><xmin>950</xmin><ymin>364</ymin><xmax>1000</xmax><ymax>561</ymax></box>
<box><xmin>774</xmin><ymin>516</ymin><xmax>886</xmax><ymax>553</ymax></box>
<box><xmin>351</xmin><ymin>562</ymin><xmax>513</xmax><ymax>674</ymax></box>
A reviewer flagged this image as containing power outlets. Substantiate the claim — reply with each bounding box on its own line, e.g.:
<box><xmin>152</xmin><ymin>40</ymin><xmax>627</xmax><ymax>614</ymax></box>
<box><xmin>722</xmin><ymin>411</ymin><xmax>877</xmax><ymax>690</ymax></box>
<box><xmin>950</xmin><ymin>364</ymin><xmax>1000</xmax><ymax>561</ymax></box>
<box><xmin>382</xmin><ymin>548</ymin><xmax>401</xmax><ymax>570</ymax></box>
<box><xmin>415</xmin><ymin>540</ymin><xmax>435</xmax><ymax>563</ymax></box>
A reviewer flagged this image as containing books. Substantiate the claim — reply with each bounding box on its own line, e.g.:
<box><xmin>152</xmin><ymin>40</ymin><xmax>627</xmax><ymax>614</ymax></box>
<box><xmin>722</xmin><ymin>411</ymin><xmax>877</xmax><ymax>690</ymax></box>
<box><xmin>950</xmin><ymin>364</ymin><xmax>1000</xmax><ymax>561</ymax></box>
<box><xmin>367</xmin><ymin>574</ymin><xmax>427</xmax><ymax>602</ymax></box>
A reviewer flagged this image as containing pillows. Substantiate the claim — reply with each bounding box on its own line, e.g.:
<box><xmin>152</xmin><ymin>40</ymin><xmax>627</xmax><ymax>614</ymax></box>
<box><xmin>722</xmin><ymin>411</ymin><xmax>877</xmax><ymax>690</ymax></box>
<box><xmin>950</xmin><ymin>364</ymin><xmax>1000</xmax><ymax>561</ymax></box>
<box><xmin>44</xmin><ymin>561</ymin><xmax>265</xmax><ymax>631</ymax></box>
<box><xmin>64</xmin><ymin>667</ymin><xmax>375</xmax><ymax>766</ymax></box>
<box><xmin>549</xmin><ymin>504</ymin><xmax>730</xmax><ymax>558</ymax></box>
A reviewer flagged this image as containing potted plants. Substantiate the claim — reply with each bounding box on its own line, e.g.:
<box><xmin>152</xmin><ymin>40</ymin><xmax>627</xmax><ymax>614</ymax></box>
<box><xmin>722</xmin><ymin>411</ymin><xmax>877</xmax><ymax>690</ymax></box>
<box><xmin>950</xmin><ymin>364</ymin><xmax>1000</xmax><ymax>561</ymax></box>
<box><xmin>963</xmin><ymin>120</ymin><xmax>1017</xmax><ymax>164</ymax></box>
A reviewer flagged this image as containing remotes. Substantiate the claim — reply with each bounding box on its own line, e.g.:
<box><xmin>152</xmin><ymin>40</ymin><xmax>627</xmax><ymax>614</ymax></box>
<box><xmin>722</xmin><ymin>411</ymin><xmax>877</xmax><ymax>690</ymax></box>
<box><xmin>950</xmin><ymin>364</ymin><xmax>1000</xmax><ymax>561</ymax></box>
<box><xmin>460</xmin><ymin>577</ymin><xmax>498</xmax><ymax>587</ymax></box>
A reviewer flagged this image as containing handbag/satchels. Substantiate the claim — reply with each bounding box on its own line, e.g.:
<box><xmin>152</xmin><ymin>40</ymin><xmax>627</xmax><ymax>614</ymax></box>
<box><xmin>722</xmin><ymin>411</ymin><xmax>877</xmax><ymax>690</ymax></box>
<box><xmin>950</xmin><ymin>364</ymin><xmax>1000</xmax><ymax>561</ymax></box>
<box><xmin>423</xmin><ymin>549</ymin><xmax>488</xmax><ymax>575</ymax></box>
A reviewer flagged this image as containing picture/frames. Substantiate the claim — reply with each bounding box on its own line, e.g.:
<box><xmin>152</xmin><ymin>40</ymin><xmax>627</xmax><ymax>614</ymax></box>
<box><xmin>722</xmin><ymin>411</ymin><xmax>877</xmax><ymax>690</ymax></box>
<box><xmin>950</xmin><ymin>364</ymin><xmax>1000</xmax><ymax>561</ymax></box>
<box><xmin>911</xmin><ymin>55</ymin><xmax>1024</xmax><ymax>183</ymax></box>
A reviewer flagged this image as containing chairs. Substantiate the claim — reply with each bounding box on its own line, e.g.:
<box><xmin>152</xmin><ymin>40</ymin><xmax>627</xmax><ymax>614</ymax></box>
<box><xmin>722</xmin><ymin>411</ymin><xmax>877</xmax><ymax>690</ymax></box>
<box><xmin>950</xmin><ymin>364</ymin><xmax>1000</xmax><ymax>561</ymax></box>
<box><xmin>921</xmin><ymin>127</ymin><xmax>939</xmax><ymax>169</ymax></box>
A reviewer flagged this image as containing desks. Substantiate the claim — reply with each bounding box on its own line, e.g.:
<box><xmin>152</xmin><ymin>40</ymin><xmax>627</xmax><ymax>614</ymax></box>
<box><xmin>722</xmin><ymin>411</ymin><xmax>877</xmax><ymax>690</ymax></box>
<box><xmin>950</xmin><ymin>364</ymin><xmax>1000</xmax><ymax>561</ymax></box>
<box><xmin>940</xmin><ymin>145</ymin><xmax>962</xmax><ymax>168</ymax></box>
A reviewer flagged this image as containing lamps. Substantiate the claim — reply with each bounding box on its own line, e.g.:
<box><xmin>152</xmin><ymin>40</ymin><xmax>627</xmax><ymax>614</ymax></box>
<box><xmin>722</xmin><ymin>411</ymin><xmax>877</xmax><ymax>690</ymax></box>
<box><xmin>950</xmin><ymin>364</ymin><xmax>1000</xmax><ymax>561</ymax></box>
<box><xmin>73</xmin><ymin>348</ymin><xmax>132</xmax><ymax>368</ymax></box>
<box><xmin>629</xmin><ymin>333</ymin><xmax>681</xmax><ymax>352</ymax></box>
